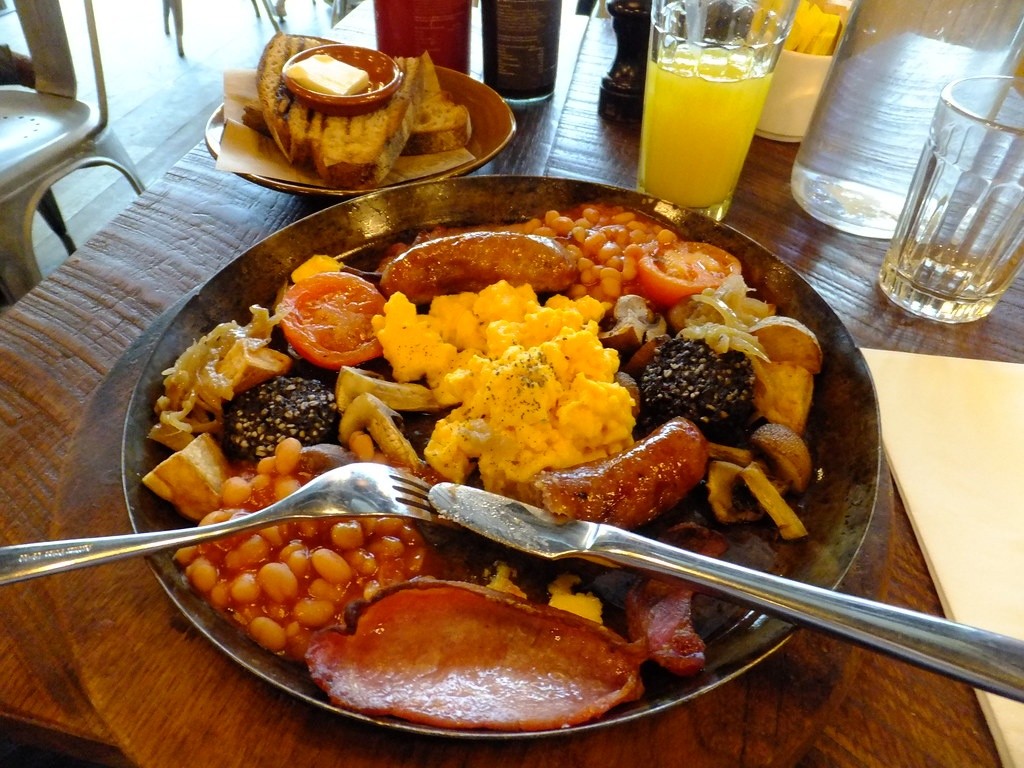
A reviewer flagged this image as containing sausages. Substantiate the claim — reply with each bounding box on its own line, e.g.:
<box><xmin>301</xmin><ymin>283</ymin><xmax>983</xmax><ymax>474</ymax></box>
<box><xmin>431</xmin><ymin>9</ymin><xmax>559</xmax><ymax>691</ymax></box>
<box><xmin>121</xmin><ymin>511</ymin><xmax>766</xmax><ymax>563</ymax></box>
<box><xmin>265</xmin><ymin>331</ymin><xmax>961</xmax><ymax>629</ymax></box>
<box><xmin>534</xmin><ymin>417</ymin><xmax>709</xmax><ymax>535</ymax></box>
<box><xmin>377</xmin><ymin>228</ymin><xmax>574</xmax><ymax>292</ymax></box>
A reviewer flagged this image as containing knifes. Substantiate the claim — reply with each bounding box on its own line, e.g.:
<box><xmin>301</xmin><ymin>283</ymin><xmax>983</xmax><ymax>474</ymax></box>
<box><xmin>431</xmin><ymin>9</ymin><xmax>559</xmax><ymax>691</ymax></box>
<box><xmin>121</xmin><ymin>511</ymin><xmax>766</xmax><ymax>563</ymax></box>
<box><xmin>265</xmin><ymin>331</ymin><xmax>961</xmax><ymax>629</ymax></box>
<box><xmin>428</xmin><ymin>483</ymin><xmax>1022</xmax><ymax>705</ymax></box>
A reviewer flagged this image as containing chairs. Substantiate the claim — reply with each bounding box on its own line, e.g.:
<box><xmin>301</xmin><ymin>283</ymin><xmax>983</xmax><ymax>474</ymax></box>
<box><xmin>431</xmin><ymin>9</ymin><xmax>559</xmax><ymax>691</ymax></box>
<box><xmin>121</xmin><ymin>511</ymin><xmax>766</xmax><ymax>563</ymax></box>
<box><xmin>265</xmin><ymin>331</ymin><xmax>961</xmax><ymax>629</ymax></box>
<box><xmin>1</xmin><ymin>1</ymin><xmax>147</xmax><ymax>307</ymax></box>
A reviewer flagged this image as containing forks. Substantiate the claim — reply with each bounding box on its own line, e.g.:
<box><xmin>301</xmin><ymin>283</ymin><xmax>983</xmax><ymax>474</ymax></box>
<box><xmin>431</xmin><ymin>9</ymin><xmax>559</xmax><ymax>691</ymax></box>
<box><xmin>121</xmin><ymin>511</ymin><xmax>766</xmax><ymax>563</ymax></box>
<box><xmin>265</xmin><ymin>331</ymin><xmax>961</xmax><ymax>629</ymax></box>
<box><xmin>0</xmin><ymin>460</ymin><xmax>466</xmax><ymax>585</ymax></box>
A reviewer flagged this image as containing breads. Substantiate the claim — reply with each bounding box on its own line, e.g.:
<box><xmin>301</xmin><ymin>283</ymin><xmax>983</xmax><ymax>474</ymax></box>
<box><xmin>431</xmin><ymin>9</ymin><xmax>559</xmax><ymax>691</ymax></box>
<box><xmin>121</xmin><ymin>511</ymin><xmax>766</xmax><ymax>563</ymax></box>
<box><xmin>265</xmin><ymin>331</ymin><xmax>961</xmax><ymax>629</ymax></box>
<box><xmin>244</xmin><ymin>30</ymin><xmax>473</xmax><ymax>185</ymax></box>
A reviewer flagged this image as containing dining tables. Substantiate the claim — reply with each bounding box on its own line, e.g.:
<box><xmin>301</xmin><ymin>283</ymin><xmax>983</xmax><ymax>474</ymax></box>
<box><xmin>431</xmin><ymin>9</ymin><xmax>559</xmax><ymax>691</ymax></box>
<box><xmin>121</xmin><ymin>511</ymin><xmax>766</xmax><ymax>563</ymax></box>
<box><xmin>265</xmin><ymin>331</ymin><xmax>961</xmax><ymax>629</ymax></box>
<box><xmin>2</xmin><ymin>1</ymin><xmax>1023</xmax><ymax>767</ymax></box>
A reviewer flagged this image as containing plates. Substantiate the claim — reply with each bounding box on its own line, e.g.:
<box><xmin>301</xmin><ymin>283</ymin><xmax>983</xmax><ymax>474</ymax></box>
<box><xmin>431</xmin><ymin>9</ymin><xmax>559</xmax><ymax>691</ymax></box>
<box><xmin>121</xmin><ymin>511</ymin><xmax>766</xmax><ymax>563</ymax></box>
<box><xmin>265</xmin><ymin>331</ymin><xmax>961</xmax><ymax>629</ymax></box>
<box><xmin>205</xmin><ymin>65</ymin><xmax>517</xmax><ymax>200</ymax></box>
<box><xmin>285</xmin><ymin>45</ymin><xmax>402</xmax><ymax>117</ymax></box>
<box><xmin>117</xmin><ymin>172</ymin><xmax>883</xmax><ymax>739</ymax></box>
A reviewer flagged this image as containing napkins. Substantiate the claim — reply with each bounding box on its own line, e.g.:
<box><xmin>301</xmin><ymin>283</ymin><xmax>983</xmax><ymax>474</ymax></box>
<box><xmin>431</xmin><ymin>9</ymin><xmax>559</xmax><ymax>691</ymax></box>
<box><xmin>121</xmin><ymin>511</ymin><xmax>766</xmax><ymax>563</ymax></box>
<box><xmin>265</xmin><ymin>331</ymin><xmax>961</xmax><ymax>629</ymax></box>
<box><xmin>859</xmin><ymin>346</ymin><xmax>1021</xmax><ymax>768</ymax></box>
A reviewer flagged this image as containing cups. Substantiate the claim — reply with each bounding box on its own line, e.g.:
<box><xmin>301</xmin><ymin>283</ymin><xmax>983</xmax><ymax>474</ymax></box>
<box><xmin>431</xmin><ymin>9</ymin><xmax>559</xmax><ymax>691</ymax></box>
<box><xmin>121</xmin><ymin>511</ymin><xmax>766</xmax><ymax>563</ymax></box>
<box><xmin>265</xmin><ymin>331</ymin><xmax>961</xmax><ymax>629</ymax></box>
<box><xmin>880</xmin><ymin>72</ymin><xmax>1021</xmax><ymax>322</ymax></box>
<box><xmin>372</xmin><ymin>2</ymin><xmax>469</xmax><ymax>75</ymax></box>
<box><xmin>751</xmin><ymin>48</ymin><xmax>838</xmax><ymax>143</ymax></box>
<box><xmin>788</xmin><ymin>2</ymin><xmax>1021</xmax><ymax>240</ymax></box>
<box><xmin>635</xmin><ymin>0</ymin><xmax>792</xmax><ymax>221</ymax></box>
<box><xmin>481</xmin><ymin>1</ymin><xmax>558</xmax><ymax>100</ymax></box>
